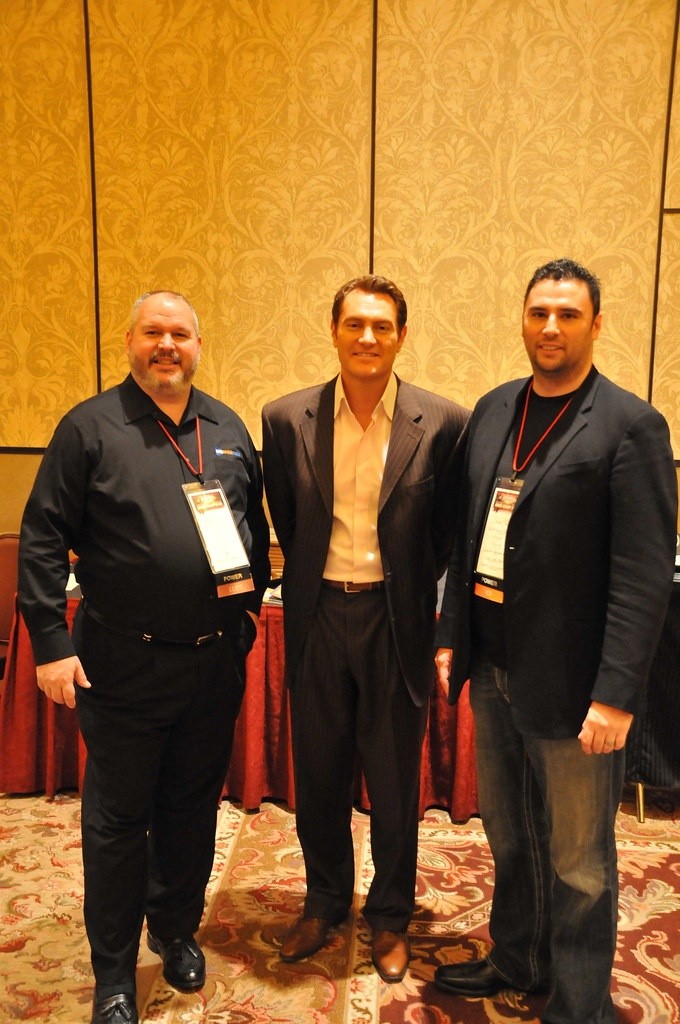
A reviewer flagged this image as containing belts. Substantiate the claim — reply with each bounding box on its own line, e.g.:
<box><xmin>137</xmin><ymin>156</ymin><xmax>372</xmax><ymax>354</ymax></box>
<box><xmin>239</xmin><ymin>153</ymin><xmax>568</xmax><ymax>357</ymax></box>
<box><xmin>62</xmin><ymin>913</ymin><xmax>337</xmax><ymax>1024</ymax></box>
<box><xmin>473</xmin><ymin>582</ymin><xmax>506</xmax><ymax>604</ymax></box>
<box><xmin>322</xmin><ymin>579</ymin><xmax>385</xmax><ymax>594</ymax></box>
<box><xmin>82</xmin><ymin>599</ymin><xmax>241</xmax><ymax>648</ymax></box>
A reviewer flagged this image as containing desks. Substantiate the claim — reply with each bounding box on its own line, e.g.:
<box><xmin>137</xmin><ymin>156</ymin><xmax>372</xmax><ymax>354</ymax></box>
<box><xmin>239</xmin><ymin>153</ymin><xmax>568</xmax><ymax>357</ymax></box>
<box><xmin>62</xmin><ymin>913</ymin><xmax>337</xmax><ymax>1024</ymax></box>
<box><xmin>0</xmin><ymin>593</ymin><xmax>481</xmax><ymax>825</ymax></box>
<box><xmin>622</xmin><ymin>566</ymin><xmax>680</xmax><ymax>824</ymax></box>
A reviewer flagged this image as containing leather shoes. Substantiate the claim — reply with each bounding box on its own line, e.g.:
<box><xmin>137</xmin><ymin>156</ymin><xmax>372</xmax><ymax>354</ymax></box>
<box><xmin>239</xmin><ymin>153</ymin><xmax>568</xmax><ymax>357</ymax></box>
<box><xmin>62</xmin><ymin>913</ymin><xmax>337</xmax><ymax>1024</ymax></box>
<box><xmin>147</xmin><ymin>927</ymin><xmax>205</xmax><ymax>990</ymax></box>
<box><xmin>280</xmin><ymin>907</ymin><xmax>350</xmax><ymax>961</ymax></box>
<box><xmin>92</xmin><ymin>984</ymin><xmax>138</xmax><ymax>1024</ymax></box>
<box><xmin>434</xmin><ymin>960</ymin><xmax>512</xmax><ymax>998</ymax></box>
<box><xmin>372</xmin><ymin>929</ymin><xmax>411</xmax><ymax>981</ymax></box>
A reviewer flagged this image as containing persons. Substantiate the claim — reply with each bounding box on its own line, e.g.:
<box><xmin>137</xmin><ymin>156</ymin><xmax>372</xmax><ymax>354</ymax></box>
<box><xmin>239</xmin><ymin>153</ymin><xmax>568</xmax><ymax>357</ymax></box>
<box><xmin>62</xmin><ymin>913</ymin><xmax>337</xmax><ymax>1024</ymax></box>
<box><xmin>18</xmin><ymin>292</ymin><xmax>272</xmax><ymax>1024</ymax></box>
<box><xmin>435</xmin><ymin>259</ymin><xmax>677</xmax><ymax>1024</ymax></box>
<box><xmin>262</xmin><ymin>274</ymin><xmax>472</xmax><ymax>977</ymax></box>
